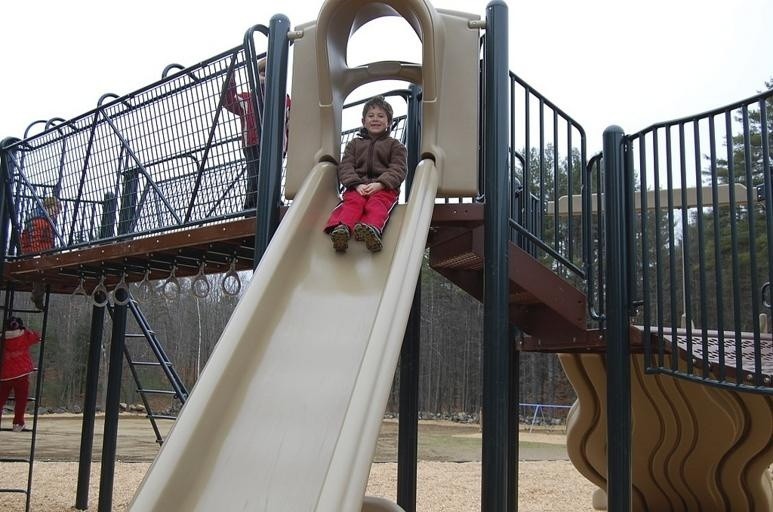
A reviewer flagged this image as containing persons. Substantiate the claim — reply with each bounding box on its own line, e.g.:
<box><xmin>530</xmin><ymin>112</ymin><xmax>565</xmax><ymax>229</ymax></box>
<box><xmin>0</xmin><ymin>317</ymin><xmax>41</xmax><ymax>433</ymax></box>
<box><xmin>322</xmin><ymin>95</ymin><xmax>409</xmax><ymax>252</ymax></box>
<box><xmin>222</xmin><ymin>57</ymin><xmax>291</xmax><ymax>219</ymax></box>
<box><xmin>20</xmin><ymin>196</ymin><xmax>62</xmax><ymax>310</ymax></box>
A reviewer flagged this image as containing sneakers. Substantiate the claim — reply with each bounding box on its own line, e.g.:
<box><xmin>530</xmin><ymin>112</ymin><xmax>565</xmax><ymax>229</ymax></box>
<box><xmin>353</xmin><ymin>223</ymin><xmax>383</xmax><ymax>251</ymax></box>
<box><xmin>12</xmin><ymin>422</ymin><xmax>26</xmax><ymax>432</ymax></box>
<box><xmin>330</xmin><ymin>224</ymin><xmax>350</xmax><ymax>251</ymax></box>
<box><xmin>30</xmin><ymin>295</ymin><xmax>43</xmax><ymax>310</ymax></box>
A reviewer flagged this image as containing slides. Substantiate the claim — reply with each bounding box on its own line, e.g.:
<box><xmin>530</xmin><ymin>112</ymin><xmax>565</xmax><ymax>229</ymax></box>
<box><xmin>125</xmin><ymin>159</ymin><xmax>440</xmax><ymax>512</ymax></box>
<box><xmin>557</xmin><ymin>351</ymin><xmax>769</xmax><ymax>512</ymax></box>
